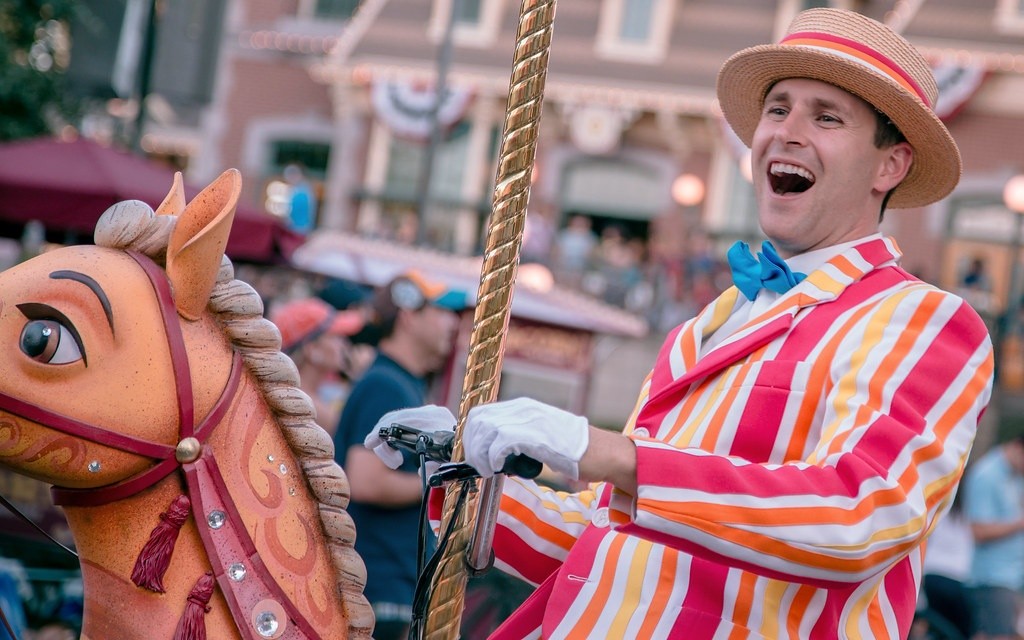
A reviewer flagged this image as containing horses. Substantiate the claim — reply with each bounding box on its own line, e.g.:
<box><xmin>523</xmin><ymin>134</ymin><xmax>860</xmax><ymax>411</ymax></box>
<box><xmin>1</xmin><ymin>167</ymin><xmax>379</xmax><ymax>640</ymax></box>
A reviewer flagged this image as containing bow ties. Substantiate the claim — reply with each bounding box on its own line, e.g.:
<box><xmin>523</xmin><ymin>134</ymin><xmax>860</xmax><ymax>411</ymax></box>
<box><xmin>726</xmin><ymin>240</ymin><xmax>808</xmax><ymax>302</ymax></box>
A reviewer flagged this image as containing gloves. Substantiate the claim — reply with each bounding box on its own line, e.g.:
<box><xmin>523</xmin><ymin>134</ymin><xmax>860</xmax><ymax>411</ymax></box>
<box><xmin>462</xmin><ymin>397</ymin><xmax>589</xmax><ymax>482</ymax></box>
<box><xmin>364</xmin><ymin>404</ymin><xmax>457</xmax><ymax>488</ymax></box>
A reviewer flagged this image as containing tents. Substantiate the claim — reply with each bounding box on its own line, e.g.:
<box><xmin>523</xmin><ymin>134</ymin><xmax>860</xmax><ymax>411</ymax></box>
<box><xmin>1</xmin><ymin>134</ymin><xmax>303</xmax><ymax>269</ymax></box>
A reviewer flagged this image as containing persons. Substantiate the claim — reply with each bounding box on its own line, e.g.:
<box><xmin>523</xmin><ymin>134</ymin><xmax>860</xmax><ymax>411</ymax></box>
<box><xmin>388</xmin><ymin>206</ymin><xmax>993</xmax><ymax>356</ymax></box>
<box><xmin>912</xmin><ymin>417</ymin><xmax>1023</xmax><ymax>639</ymax></box>
<box><xmin>267</xmin><ymin>272</ymin><xmax>473</xmax><ymax>637</ymax></box>
<box><xmin>364</xmin><ymin>9</ymin><xmax>993</xmax><ymax>639</ymax></box>
<box><xmin>282</xmin><ymin>167</ymin><xmax>315</xmax><ymax>234</ymax></box>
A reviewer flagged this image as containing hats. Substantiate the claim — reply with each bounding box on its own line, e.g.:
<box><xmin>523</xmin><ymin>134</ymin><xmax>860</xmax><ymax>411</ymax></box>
<box><xmin>376</xmin><ymin>269</ymin><xmax>472</xmax><ymax>319</ymax></box>
<box><xmin>717</xmin><ymin>7</ymin><xmax>963</xmax><ymax>209</ymax></box>
